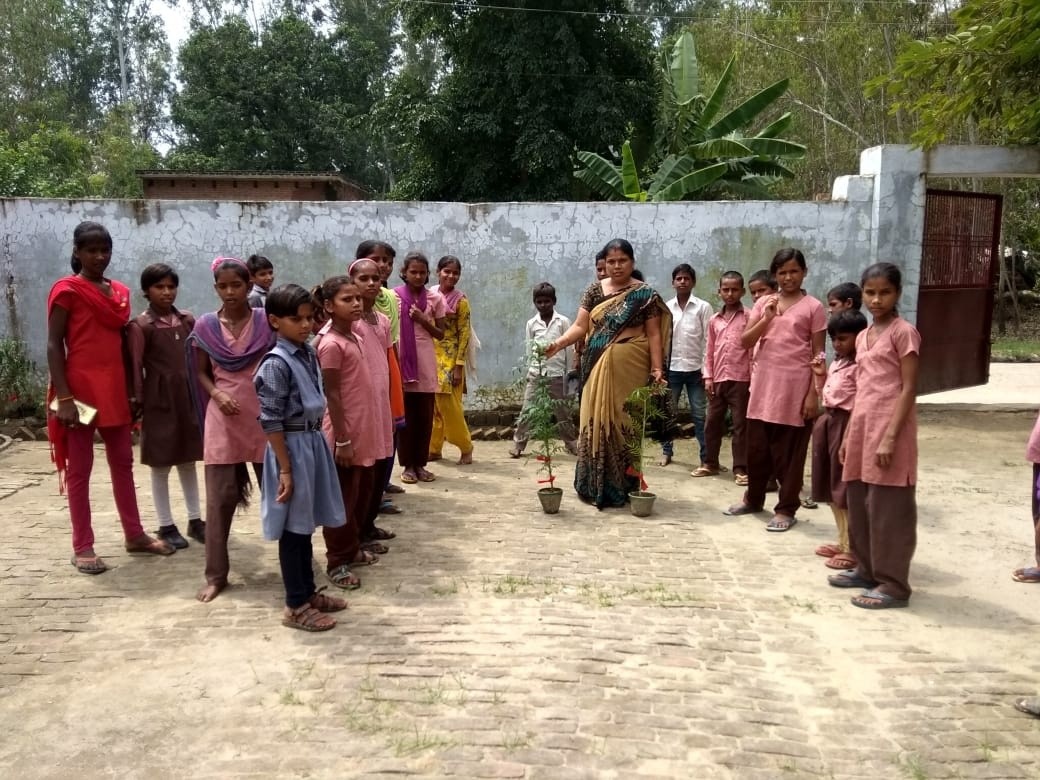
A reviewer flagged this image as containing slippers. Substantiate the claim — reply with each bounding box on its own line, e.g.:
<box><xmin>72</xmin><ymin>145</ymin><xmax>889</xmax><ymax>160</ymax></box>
<box><xmin>690</xmin><ymin>465</ymin><xmax>720</xmax><ymax>476</ymax></box>
<box><xmin>127</xmin><ymin>538</ymin><xmax>177</xmax><ymax>555</ymax></box>
<box><xmin>801</xmin><ymin>495</ymin><xmax>819</xmax><ymax>508</ymax></box>
<box><xmin>71</xmin><ymin>555</ymin><xmax>106</xmax><ymax>574</ymax></box>
<box><xmin>765</xmin><ymin>517</ymin><xmax>797</xmax><ymax>531</ymax></box>
<box><xmin>851</xmin><ymin>590</ymin><xmax>908</xmax><ymax>609</ymax></box>
<box><xmin>827</xmin><ymin>570</ymin><xmax>867</xmax><ymax>588</ymax></box>
<box><xmin>412</xmin><ymin>467</ymin><xmax>436</xmax><ymax>481</ymax></box>
<box><xmin>824</xmin><ymin>554</ymin><xmax>858</xmax><ymax>569</ymax></box>
<box><xmin>735</xmin><ymin>474</ymin><xmax>748</xmax><ymax>485</ymax></box>
<box><xmin>723</xmin><ymin>503</ymin><xmax>763</xmax><ymax>516</ymax></box>
<box><xmin>816</xmin><ymin>544</ymin><xmax>842</xmax><ymax>556</ymax></box>
<box><xmin>401</xmin><ymin>470</ymin><xmax>418</xmax><ymax>484</ymax></box>
<box><xmin>1012</xmin><ymin>567</ymin><xmax>1040</xmax><ymax>583</ymax></box>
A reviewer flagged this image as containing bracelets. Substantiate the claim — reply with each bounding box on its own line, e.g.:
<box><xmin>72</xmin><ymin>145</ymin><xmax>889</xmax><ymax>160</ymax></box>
<box><xmin>59</xmin><ymin>394</ymin><xmax>74</xmax><ymax>401</ymax></box>
<box><xmin>213</xmin><ymin>391</ymin><xmax>225</xmax><ymax>399</ymax></box>
<box><xmin>652</xmin><ymin>368</ymin><xmax>662</xmax><ymax>372</ymax></box>
<box><xmin>279</xmin><ymin>469</ymin><xmax>291</xmax><ymax>475</ymax></box>
<box><xmin>336</xmin><ymin>440</ymin><xmax>351</xmax><ymax>447</ymax></box>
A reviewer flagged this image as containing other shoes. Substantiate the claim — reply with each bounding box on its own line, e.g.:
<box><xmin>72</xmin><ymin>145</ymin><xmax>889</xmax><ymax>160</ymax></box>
<box><xmin>380</xmin><ymin>491</ymin><xmax>394</xmax><ymax>501</ymax></box>
<box><xmin>153</xmin><ymin>525</ymin><xmax>188</xmax><ymax>549</ymax></box>
<box><xmin>378</xmin><ymin>501</ymin><xmax>403</xmax><ymax>513</ymax></box>
<box><xmin>187</xmin><ymin>519</ymin><xmax>206</xmax><ymax>544</ymax></box>
<box><xmin>388</xmin><ymin>482</ymin><xmax>407</xmax><ymax>493</ymax></box>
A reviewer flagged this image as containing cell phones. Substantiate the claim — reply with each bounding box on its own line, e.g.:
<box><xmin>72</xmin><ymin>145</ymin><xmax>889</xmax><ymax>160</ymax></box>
<box><xmin>647</xmin><ymin>376</ymin><xmax>669</xmax><ymax>393</ymax></box>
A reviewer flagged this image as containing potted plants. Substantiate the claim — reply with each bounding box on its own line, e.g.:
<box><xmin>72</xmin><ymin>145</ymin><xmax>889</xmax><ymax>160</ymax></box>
<box><xmin>510</xmin><ymin>343</ymin><xmax>573</xmax><ymax>512</ymax></box>
<box><xmin>622</xmin><ymin>384</ymin><xmax>668</xmax><ymax>516</ymax></box>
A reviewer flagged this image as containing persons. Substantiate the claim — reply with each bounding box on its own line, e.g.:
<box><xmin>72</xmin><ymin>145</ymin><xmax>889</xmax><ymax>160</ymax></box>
<box><xmin>45</xmin><ymin>222</ymin><xmax>178</xmax><ymax>573</ymax></box>
<box><xmin>128</xmin><ymin>241</ymin><xmax>474</xmax><ymax>632</ymax></box>
<box><xmin>508</xmin><ymin>242</ymin><xmax>1040</xmax><ymax>717</ymax></box>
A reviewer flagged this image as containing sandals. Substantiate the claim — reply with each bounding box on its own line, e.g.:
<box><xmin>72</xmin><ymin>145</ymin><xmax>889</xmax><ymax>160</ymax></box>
<box><xmin>360</xmin><ymin>541</ymin><xmax>389</xmax><ymax>557</ymax></box>
<box><xmin>311</xmin><ymin>584</ymin><xmax>346</xmax><ymax>611</ymax></box>
<box><xmin>327</xmin><ymin>565</ymin><xmax>360</xmax><ymax>589</ymax></box>
<box><xmin>346</xmin><ymin>549</ymin><xmax>380</xmax><ymax>568</ymax></box>
<box><xmin>370</xmin><ymin>526</ymin><xmax>396</xmax><ymax>541</ymax></box>
<box><xmin>281</xmin><ymin>604</ymin><xmax>336</xmax><ymax>632</ymax></box>
